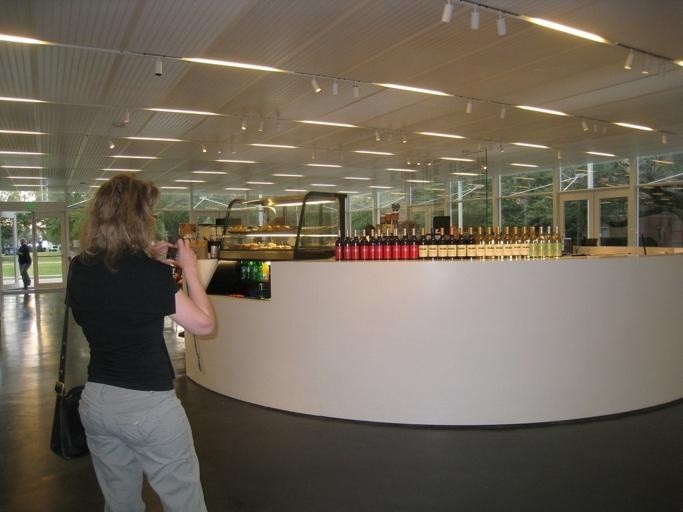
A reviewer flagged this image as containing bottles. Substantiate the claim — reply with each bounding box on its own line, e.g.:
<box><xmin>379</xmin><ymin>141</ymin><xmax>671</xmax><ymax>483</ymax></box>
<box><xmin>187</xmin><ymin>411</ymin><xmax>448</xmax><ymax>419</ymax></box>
<box><xmin>240</xmin><ymin>261</ymin><xmax>269</xmax><ymax>281</ymax></box>
<box><xmin>334</xmin><ymin>224</ymin><xmax>562</xmax><ymax>260</ymax></box>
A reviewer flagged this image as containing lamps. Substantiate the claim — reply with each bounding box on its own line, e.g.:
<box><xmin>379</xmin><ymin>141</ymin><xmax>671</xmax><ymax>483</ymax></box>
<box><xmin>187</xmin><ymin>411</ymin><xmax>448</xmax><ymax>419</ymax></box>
<box><xmin>616</xmin><ymin>42</ymin><xmax>674</xmax><ymax>78</ymax></box>
<box><xmin>575</xmin><ymin>116</ymin><xmax>610</xmax><ymax>134</ymax></box>
<box><xmin>198</xmin><ymin>140</ymin><xmax>236</xmax><ymax>155</ymax></box>
<box><xmin>107</xmin><ymin>139</ymin><xmax>115</xmax><ymax>149</ymax></box>
<box><xmin>375</xmin><ymin>129</ymin><xmax>381</xmax><ymax>142</ymax></box>
<box><xmin>293</xmin><ymin>72</ymin><xmax>367</xmax><ymax>99</ymax></box>
<box><xmin>489</xmin><ymin>100</ymin><xmax>513</xmax><ymax>118</ymax></box>
<box><xmin>124</xmin><ymin>111</ymin><xmax>129</xmax><ymax>123</ymax></box>
<box><xmin>442</xmin><ymin>0</ymin><xmax>519</xmax><ymax>36</ymax></box>
<box><xmin>662</xmin><ymin>132</ymin><xmax>667</xmax><ymax>144</ymax></box>
<box><xmin>455</xmin><ymin>95</ymin><xmax>483</xmax><ymax>114</ymax></box>
<box><xmin>235</xmin><ymin>113</ymin><xmax>284</xmax><ymax>132</ymax></box>
<box><xmin>141</xmin><ymin>53</ymin><xmax>181</xmax><ymax>76</ymax></box>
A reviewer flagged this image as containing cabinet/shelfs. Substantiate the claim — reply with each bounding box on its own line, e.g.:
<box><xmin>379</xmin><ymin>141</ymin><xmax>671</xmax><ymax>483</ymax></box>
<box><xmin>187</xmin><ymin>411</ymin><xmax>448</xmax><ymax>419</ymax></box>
<box><xmin>215</xmin><ymin>192</ymin><xmax>347</xmax><ymax>261</ymax></box>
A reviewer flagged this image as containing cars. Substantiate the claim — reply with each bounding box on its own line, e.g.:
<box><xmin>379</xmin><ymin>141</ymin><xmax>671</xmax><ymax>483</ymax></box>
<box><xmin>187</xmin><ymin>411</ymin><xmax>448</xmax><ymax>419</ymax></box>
<box><xmin>3</xmin><ymin>240</ymin><xmax>60</xmax><ymax>255</ymax></box>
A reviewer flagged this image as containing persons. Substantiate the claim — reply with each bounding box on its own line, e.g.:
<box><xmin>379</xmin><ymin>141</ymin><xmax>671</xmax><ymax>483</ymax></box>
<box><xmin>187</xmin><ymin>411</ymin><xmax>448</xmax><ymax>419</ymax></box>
<box><xmin>15</xmin><ymin>239</ymin><xmax>32</xmax><ymax>288</ymax></box>
<box><xmin>65</xmin><ymin>172</ymin><xmax>217</xmax><ymax>512</ymax></box>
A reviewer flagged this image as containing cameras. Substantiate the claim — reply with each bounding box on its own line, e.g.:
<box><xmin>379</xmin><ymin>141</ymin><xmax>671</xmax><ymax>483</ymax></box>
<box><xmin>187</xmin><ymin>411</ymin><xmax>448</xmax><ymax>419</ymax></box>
<box><xmin>166</xmin><ymin>247</ymin><xmax>177</xmax><ymax>260</ymax></box>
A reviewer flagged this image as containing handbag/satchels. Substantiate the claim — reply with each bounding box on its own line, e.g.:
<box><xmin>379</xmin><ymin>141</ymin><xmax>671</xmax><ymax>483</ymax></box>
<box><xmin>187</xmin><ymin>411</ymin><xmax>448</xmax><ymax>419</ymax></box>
<box><xmin>50</xmin><ymin>385</ymin><xmax>90</xmax><ymax>461</ymax></box>
